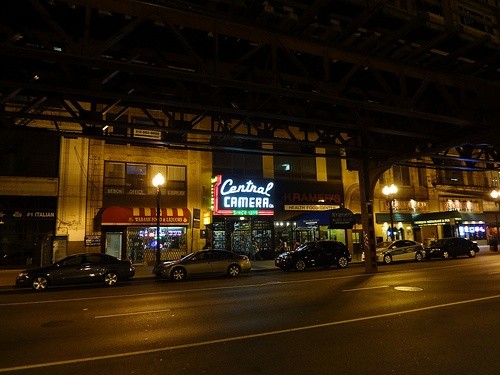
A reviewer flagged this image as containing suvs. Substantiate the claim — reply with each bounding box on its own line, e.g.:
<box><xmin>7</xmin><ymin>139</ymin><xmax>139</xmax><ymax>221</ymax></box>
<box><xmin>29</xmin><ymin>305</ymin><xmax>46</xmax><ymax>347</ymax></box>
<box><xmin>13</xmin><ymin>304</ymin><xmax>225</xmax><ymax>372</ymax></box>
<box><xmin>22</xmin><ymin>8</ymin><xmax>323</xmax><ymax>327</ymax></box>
<box><xmin>274</xmin><ymin>240</ymin><xmax>352</xmax><ymax>272</ymax></box>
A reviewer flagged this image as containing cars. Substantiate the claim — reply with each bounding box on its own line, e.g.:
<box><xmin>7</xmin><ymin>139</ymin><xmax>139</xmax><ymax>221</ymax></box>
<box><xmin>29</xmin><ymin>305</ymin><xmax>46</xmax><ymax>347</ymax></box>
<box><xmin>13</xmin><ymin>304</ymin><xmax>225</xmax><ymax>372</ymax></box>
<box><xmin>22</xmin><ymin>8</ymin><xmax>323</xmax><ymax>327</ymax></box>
<box><xmin>361</xmin><ymin>240</ymin><xmax>423</xmax><ymax>265</ymax></box>
<box><xmin>16</xmin><ymin>253</ymin><xmax>136</xmax><ymax>292</ymax></box>
<box><xmin>152</xmin><ymin>249</ymin><xmax>252</xmax><ymax>282</ymax></box>
<box><xmin>425</xmin><ymin>237</ymin><xmax>479</xmax><ymax>261</ymax></box>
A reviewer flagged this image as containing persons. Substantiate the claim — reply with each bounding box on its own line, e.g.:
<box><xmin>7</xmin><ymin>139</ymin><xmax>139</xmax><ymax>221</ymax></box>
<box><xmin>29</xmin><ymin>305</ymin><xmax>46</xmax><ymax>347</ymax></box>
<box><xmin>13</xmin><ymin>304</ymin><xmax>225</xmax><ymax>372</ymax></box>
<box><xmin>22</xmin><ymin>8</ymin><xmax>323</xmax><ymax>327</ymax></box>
<box><xmin>253</xmin><ymin>240</ymin><xmax>263</xmax><ymax>260</ymax></box>
<box><xmin>293</xmin><ymin>235</ymin><xmax>337</xmax><ymax>251</ymax></box>
<box><xmin>277</xmin><ymin>237</ymin><xmax>285</xmax><ymax>253</ymax></box>
<box><xmin>430</xmin><ymin>232</ymin><xmax>438</xmax><ymax>242</ymax></box>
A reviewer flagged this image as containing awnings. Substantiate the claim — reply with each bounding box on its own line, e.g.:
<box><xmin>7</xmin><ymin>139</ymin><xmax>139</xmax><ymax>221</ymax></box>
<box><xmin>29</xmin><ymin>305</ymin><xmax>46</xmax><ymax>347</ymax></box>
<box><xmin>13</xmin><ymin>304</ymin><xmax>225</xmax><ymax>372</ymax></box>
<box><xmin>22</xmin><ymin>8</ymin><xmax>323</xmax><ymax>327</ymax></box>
<box><xmin>291</xmin><ymin>208</ymin><xmax>354</xmax><ymax>229</ymax></box>
<box><xmin>97</xmin><ymin>205</ymin><xmax>191</xmax><ymax>228</ymax></box>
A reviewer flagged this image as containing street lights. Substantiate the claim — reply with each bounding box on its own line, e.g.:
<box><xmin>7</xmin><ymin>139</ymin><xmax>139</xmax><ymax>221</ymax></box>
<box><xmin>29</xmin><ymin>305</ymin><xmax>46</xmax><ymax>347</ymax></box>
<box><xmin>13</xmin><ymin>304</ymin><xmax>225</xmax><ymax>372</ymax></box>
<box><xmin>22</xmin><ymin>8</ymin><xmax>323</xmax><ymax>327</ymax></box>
<box><xmin>152</xmin><ymin>173</ymin><xmax>164</xmax><ymax>262</ymax></box>
<box><xmin>491</xmin><ymin>189</ymin><xmax>499</xmax><ymax>235</ymax></box>
<box><xmin>383</xmin><ymin>184</ymin><xmax>397</xmax><ymax>241</ymax></box>
